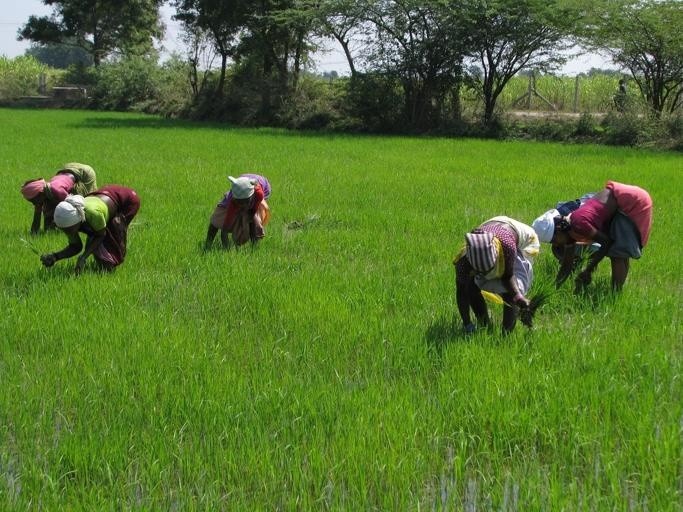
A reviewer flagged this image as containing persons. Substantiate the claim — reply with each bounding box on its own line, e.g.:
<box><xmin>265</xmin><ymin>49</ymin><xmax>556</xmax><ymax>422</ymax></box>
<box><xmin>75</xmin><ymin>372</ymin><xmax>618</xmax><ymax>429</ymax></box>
<box><xmin>206</xmin><ymin>174</ymin><xmax>271</xmax><ymax>249</ymax></box>
<box><xmin>619</xmin><ymin>80</ymin><xmax>626</xmax><ymax>94</ymax></box>
<box><xmin>41</xmin><ymin>184</ymin><xmax>140</xmax><ymax>273</ymax></box>
<box><xmin>453</xmin><ymin>216</ymin><xmax>540</xmax><ymax>337</ymax></box>
<box><xmin>531</xmin><ymin>180</ymin><xmax>653</xmax><ymax>293</ymax></box>
<box><xmin>21</xmin><ymin>162</ymin><xmax>97</xmax><ymax>233</ymax></box>
